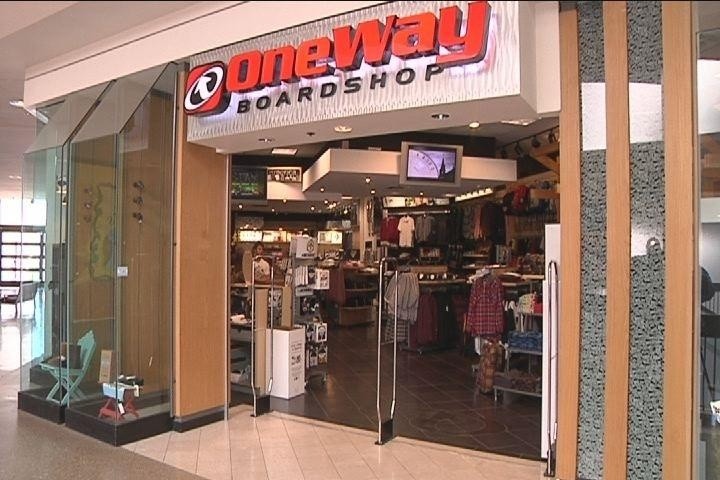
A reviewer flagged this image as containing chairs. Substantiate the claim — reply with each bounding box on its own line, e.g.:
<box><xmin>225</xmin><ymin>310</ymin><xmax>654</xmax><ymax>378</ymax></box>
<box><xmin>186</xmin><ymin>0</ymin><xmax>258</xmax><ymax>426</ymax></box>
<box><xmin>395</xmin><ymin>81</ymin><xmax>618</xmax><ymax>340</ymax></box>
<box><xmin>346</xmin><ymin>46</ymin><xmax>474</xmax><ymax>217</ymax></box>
<box><xmin>38</xmin><ymin>330</ymin><xmax>96</xmax><ymax>407</ymax></box>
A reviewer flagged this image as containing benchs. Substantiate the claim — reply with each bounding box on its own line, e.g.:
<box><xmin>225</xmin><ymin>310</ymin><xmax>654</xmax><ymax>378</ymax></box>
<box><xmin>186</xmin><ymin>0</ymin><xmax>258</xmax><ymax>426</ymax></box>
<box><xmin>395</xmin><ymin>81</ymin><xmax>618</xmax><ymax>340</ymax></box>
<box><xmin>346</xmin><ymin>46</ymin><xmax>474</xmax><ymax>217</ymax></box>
<box><xmin>0</xmin><ymin>283</ymin><xmax>37</xmax><ymax>320</ymax></box>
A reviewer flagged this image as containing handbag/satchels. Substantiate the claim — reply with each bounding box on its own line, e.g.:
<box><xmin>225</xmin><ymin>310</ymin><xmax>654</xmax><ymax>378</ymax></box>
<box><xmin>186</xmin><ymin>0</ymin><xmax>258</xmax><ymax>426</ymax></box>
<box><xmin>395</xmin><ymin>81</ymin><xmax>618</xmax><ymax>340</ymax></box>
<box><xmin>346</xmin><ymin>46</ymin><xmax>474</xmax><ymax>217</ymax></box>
<box><xmin>517</xmin><ymin>292</ymin><xmax>536</xmax><ymax>316</ymax></box>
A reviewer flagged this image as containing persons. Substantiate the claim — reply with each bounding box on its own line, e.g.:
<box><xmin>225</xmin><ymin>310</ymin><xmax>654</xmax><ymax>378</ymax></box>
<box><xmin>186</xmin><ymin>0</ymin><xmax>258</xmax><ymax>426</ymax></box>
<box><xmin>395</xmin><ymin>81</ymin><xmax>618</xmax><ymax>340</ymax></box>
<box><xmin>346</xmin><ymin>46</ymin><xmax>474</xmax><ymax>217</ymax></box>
<box><xmin>251</xmin><ymin>241</ymin><xmax>270</xmax><ymax>284</ymax></box>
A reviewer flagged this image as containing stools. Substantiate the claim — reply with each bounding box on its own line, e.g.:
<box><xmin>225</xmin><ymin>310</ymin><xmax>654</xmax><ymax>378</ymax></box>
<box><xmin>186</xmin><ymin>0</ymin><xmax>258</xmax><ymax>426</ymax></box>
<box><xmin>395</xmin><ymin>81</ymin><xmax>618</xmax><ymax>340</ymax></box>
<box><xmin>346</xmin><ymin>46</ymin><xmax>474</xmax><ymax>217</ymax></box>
<box><xmin>99</xmin><ymin>383</ymin><xmax>139</xmax><ymax>421</ymax></box>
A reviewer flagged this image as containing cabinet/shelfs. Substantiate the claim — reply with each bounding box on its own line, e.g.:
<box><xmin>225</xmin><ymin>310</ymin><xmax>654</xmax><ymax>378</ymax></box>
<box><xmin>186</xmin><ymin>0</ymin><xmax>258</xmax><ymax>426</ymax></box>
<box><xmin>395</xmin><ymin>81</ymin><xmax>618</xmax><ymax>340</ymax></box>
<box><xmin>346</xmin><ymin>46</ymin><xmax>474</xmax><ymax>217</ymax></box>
<box><xmin>492</xmin><ymin>311</ymin><xmax>542</xmax><ymax>401</ymax></box>
<box><xmin>334</xmin><ymin>271</ymin><xmax>379</xmax><ymax>325</ymax></box>
<box><xmin>232</xmin><ymin>284</ymin><xmax>292</xmax><ymax>398</ymax></box>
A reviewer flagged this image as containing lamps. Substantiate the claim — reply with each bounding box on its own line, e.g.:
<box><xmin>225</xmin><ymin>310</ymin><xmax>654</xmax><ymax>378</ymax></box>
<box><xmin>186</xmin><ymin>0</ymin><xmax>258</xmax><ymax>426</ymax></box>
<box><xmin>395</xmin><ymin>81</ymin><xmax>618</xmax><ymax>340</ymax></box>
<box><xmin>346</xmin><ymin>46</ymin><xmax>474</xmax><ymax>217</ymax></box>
<box><xmin>500</xmin><ymin>128</ymin><xmax>556</xmax><ymax>159</ymax></box>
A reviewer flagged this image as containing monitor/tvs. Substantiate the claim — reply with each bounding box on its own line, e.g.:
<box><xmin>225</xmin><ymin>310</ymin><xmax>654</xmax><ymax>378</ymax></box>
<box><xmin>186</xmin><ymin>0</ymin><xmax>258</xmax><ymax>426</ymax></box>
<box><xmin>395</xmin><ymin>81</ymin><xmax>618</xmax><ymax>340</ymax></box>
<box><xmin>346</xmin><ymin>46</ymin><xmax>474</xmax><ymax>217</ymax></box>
<box><xmin>399</xmin><ymin>141</ymin><xmax>464</xmax><ymax>188</ymax></box>
<box><xmin>231</xmin><ymin>165</ymin><xmax>268</xmax><ymax>206</ymax></box>
